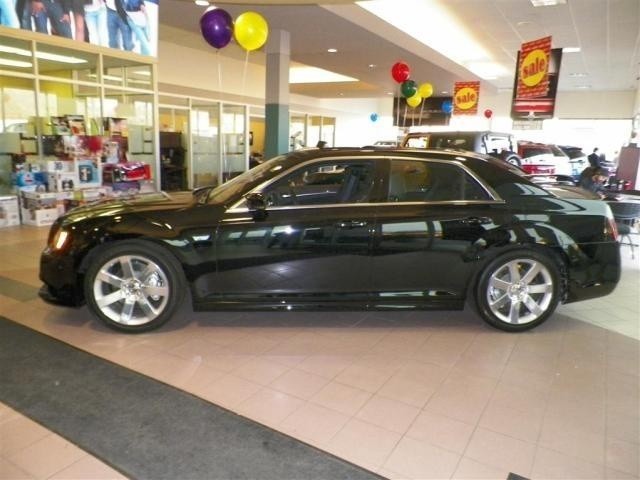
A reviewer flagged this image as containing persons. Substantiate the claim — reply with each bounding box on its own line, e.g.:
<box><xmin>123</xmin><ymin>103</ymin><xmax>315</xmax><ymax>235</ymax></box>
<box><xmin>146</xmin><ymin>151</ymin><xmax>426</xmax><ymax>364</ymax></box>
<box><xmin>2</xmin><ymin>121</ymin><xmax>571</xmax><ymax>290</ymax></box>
<box><xmin>587</xmin><ymin>147</ymin><xmax>601</xmax><ymax>167</ymax></box>
<box><xmin>13</xmin><ymin>0</ymin><xmax>150</xmax><ymax>57</ymax></box>
<box><xmin>577</xmin><ymin>166</ymin><xmax>610</xmax><ymax>200</ymax></box>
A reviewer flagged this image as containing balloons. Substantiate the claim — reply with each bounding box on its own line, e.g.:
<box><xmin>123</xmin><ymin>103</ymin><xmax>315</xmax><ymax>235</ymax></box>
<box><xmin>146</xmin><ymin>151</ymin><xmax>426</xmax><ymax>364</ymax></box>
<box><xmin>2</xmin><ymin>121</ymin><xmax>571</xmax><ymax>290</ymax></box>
<box><xmin>419</xmin><ymin>82</ymin><xmax>433</xmax><ymax>98</ymax></box>
<box><xmin>441</xmin><ymin>100</ymin><xmax>454</xmax><ymax>113</ymax></box>
<box><xmin>234</xmin><ymin>11</ymin><xmax>269</xmax><ymax>52</ymax></box>
<box><xmin>406</xmin><ymin>91</ymin><xmax>422</xmax><ymax>108</ymax></box>
<box><xmin>400</xmin><ymin>79</ymin><xmax>417</xmax><ymax>98</ymax></box>
<box><xmin>391</xmin><ymin>62</ymin><xmax>410</xmax><ymax>84</ymax></box>
<box><xmin>370</xmin><ymin>113</ymin><xmax>377</xmax><ymax>122</ymax></box>
<box><xmin>484</xmin><ymin>110</ymin><xmax>492</xmax><ymax>119</ymax></box>
<box><xmin>200</xmin><ymin>5</ymin><xmax>234</xmax><ymax>53</ymax></box>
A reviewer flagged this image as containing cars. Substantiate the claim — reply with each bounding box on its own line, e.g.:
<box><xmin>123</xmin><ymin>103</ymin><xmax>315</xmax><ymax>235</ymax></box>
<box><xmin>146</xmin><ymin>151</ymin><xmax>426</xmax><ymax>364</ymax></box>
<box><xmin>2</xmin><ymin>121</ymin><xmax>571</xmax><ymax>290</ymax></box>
<box><xmin>300</xmin><ymin>130</ymin><xmax>618</xmax><ymax>192</ymax></box>
<box><xmin>39</xmin><ymin>144</ymin><xmax>623</xmax><ymax>334</ymax></box>
<box><xmin>0</xmin><ymin>122</ymin><xmax>32</xmax><ymax>184</ymax></box>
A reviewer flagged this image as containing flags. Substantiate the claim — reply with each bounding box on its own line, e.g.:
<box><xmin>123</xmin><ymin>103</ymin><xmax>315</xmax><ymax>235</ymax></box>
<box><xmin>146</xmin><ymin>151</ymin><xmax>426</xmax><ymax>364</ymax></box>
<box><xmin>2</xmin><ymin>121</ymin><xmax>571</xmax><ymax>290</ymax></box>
<box><xmin>453</xmin><ymin>81</ymin><xmax>480</xmax><ymax>115</ymax></box>
<box><xmin>516</xmin><ymin>35</ymin><xmax>553</xmax><ymax>99</ymax></box>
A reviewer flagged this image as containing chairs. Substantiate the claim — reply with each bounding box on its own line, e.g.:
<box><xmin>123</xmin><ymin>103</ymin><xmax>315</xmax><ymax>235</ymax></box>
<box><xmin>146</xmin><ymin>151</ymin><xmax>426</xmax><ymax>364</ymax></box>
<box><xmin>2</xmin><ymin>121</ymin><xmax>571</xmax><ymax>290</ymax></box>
<box><xmin>607</xmin><ymin>201</ymin><xmax>640</xmax><ymax>261</ymax></box>
<box><xmin>334</xmin><ymin>166</ymin><xmax>361</xmax><ymax>204</ymax></box>
<box><xmin>424</xmin><ymin>168</ymin><xmax>447</xmax><ymax>201</ymax></box>
